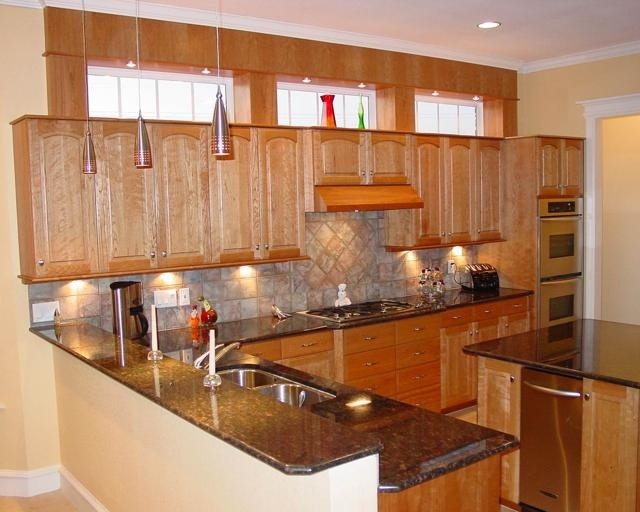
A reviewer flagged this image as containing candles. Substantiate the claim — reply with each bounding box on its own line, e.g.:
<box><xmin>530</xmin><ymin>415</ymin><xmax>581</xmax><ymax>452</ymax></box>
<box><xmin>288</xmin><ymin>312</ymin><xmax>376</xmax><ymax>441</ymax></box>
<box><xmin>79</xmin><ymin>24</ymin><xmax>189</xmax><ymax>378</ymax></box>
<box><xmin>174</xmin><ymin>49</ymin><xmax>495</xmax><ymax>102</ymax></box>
<box><xmin>209</xmin><ymin>329</ymin><xmax>215</xmax><ymax>376</ymax></box>
<box><xmin>151</xmin><ymin>305</ymin><xmax>158</xmax><ymax>352</ymax></box>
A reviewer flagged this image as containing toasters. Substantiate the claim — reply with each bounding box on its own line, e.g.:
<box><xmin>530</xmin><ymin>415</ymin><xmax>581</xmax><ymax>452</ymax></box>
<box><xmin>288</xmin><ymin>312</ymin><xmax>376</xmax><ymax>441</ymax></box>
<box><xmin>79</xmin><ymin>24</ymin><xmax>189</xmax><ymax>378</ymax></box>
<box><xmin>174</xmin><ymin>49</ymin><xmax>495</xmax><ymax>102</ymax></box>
<box><xmin>458</xmin><ymin>263</ymin><xmax>500</xmax><ymax>292</ymax></box>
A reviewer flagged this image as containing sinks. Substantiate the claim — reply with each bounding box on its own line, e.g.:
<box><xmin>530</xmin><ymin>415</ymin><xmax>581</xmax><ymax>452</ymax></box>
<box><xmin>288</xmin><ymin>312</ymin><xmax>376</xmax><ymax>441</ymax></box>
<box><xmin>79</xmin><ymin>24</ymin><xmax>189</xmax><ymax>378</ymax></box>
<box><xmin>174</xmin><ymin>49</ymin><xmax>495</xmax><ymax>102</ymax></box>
<box><xmin>248</xmin><ymin>382</ymin><xmax>338</xmax><ymax>407</ymax></box>
<box><xmin>214</xmin><ymin>366</ymin><xmax>284</xmax><ymax>391</ymax></box>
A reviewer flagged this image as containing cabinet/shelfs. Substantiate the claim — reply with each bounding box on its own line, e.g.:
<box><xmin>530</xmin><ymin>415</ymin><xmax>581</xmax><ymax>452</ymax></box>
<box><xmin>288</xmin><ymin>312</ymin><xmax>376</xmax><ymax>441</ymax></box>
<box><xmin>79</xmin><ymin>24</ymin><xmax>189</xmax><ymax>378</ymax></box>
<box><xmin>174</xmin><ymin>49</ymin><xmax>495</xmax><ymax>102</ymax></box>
<box><xmin>335</xmin><ymin>321</ymin><xmax>397</xmax><ymax>404</ymax></box>
<box><xmin>232</xmin><ymin>329</ymin><xmax>335</xmax><ymax>380</ymax></box>
<box><xmin>580</xmin><ymin>379</ymin><xmax>638</xmax><ymax>512</ymax></box>
<box><xmin>305</xmin><ymin>126</ymin><xmax>411</xmax><ymax>186</ymax></box>
<box><xmin>474</xmin><ymin>357</ymin><xmax>521</xmax><ymax>511</ymax></box>
<box><xmin>217</xmin><ymin>126</ymin><xmax>305</xmax><ymax>268</ymax></box>
<box><xmin>395</xmin><ymin>312</ymin><xmax>441</xmax><ymax>415</ymax></box>
<box><xmin>9</xmin><ymin>114</ymin><xmax>98</xmax><ymax>284</ymax></box>
<box><xmin>386</xmin><ymin>132</ymin><xmax>472</xmax><ymax>252</ymax></box>
<box><xmin>502</xmin><ymin>137</ymin><xmax>583</xmax><ymax>198</ymax></box>
<box><xmin>92</xmin><ymin>117</ymin><xmax>212</xmax><ymax>278</ymax></box>
<box><xmin>470</xmin><ymin>135</ymin><xmax>506</xmax><ymax>245</ymax></box>
<box><xmin>497</xmin><ymin>295</ymin><xmax>534</xmax><ymax>339</ymax></box>
<box><xmin>440</xmin><ymin>302</ymin><xmax>499</xmax><ymax>416</ymax></box>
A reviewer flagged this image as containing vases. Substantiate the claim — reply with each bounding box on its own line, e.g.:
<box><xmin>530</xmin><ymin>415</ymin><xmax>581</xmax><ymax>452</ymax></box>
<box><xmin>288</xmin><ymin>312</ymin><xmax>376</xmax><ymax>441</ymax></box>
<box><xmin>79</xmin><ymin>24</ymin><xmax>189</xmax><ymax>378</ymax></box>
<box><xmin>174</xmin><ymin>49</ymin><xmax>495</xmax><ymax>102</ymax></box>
<box><xmin>358</xmin><ymin>93</ymin><xmax>367</xmax><ymax>130</ymax></box>
<box><xmin>320</xmin><ymin>94</ymin><xmax>337</xmax><ymax>128</ymax></box>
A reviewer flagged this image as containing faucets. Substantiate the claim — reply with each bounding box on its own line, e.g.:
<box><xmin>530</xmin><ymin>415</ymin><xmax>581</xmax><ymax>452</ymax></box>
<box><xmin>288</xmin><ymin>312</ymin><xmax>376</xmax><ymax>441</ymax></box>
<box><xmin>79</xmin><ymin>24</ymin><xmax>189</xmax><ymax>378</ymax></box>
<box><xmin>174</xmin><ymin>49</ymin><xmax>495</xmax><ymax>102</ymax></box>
<box><xmin>191</xmin><ymin>340</ymin><xmax>243</xmax><ymax>372</ymax></box>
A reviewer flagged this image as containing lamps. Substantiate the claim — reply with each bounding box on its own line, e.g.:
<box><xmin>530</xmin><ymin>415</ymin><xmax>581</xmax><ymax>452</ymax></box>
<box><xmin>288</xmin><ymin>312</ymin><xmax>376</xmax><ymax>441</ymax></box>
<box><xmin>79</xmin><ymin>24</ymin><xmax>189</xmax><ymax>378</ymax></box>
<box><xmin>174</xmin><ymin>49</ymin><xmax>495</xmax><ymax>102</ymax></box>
<box><xmin>82</xmin><ymin>1</ymin><xmax>96</xmax><ymax>174</ymax></box>
<box><xmin>134</xmin><ymin>1</ymin><xmax>152</xmax><ymax>169</ymax></box>
<box><xmin>212</xmin><ymin>1</ymin><xmax>231</xmax><ymax>156</ymax></box>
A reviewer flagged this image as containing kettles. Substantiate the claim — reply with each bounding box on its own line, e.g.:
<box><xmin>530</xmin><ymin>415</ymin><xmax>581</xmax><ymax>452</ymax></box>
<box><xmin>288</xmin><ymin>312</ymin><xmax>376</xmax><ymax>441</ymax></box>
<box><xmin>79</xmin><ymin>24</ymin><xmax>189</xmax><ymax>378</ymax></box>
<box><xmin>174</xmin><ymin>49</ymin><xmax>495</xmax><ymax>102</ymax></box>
<box><xmin>109</xmin><ymin>280</ymin><xmax>149</xmax><ymax>342</ymax></box>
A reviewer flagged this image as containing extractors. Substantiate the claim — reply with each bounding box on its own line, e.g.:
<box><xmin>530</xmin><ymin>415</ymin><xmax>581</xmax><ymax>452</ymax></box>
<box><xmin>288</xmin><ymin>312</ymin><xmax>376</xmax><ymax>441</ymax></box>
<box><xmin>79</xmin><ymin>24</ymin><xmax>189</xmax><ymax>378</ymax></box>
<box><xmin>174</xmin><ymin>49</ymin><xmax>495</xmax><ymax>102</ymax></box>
<box><xmin>314</xmin><ymin>184</ymin><xmax>428</xmax><ymax>213</ymax></box>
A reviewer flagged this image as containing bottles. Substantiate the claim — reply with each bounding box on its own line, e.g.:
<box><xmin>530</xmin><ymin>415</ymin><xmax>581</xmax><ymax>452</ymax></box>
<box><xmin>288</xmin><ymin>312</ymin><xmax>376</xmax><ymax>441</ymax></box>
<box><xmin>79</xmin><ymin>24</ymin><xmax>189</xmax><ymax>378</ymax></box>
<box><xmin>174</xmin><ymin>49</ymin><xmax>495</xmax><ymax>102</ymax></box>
<box><xmin>197</xmin><ymin>295</ymin><xmax>218</xmax><ymax>327</ymax></box>
<box><xmin>189</xmin><ymin>304</ymin><xmax>199</xmax><ymax>329</ymax></box>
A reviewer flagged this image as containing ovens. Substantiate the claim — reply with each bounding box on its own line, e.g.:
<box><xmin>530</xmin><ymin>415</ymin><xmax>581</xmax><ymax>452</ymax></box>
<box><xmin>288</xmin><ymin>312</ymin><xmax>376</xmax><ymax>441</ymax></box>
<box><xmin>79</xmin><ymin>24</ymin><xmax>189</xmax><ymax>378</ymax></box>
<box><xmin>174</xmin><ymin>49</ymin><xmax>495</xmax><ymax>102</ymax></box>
<box><xmin>540</xmin><ymin>326</ymin><xmax>582</xmax><ymax>372</ymax></box>
<box><xmin>538</xmin><ymin>217</ymin><xmax>584</xmax><ymax>326</ymax></box>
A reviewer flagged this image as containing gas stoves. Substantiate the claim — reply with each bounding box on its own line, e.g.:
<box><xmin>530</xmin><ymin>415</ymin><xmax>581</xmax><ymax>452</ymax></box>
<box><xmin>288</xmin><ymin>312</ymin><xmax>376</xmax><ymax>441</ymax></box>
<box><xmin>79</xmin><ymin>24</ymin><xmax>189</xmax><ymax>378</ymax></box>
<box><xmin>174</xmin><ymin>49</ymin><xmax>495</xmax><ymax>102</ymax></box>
<box><xmin>300</xmin><ymin>298</ymin><xmax>433</xmax><ymax>324</ymax></box>
